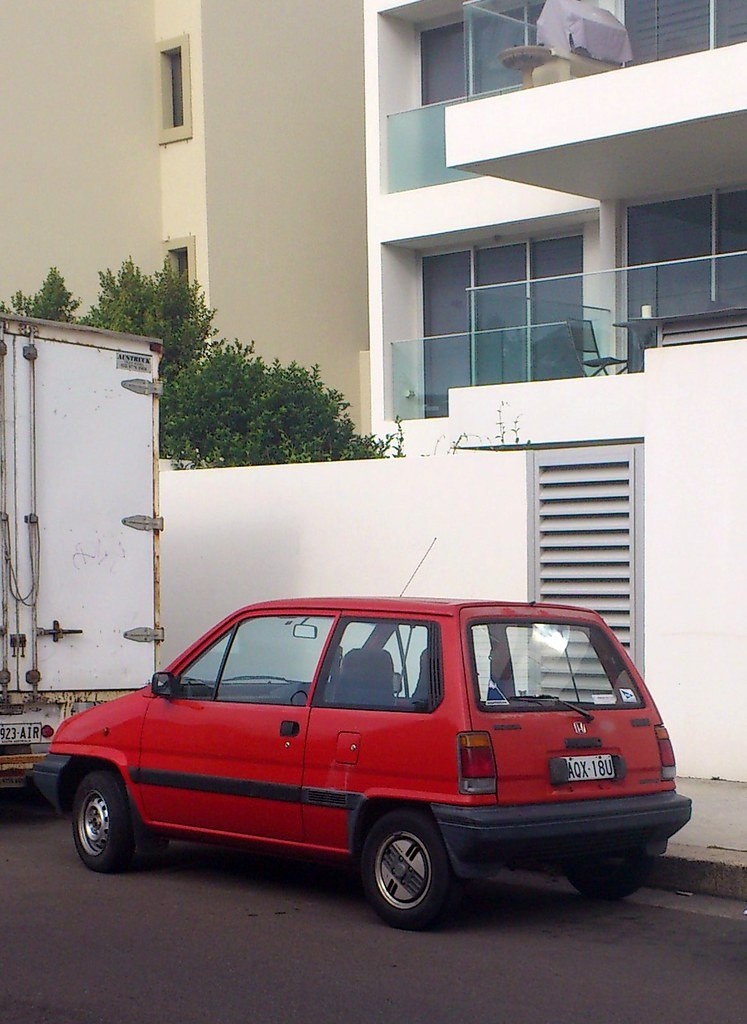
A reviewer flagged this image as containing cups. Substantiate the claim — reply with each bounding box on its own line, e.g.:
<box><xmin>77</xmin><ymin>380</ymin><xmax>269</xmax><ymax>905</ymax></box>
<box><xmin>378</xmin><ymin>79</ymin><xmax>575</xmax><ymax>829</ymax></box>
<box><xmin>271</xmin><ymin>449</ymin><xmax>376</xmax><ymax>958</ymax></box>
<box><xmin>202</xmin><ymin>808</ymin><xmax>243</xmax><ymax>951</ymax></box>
<box><xmin>642</xmin><ymin>304</ymin><xmax>652</xmax><ymax>318</ymax></box>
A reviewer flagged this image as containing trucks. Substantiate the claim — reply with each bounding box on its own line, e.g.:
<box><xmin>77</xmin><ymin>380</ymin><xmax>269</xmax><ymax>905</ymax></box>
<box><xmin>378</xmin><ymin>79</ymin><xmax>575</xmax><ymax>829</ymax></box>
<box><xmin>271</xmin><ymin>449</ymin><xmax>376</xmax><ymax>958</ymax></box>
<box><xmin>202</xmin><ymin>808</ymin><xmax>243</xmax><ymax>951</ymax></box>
<box><xmin>1</xmin><ymin>314</ymin><xmax>165</xmax><ymax>797</ymax></box>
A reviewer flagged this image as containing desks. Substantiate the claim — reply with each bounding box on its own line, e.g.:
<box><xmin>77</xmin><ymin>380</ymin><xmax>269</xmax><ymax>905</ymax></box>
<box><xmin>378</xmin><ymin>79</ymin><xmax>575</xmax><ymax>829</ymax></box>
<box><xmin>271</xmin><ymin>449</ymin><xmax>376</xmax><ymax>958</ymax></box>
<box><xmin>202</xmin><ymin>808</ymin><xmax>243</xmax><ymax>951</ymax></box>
<box><xmin>613</xmin><ymin>321</ymin><xmax>660</xmax><ymax>373</ymax></box>
<box><xmin>532</xmin><ymin>46</ymin><xmax>623</xmax><ymax>86</ymax></box>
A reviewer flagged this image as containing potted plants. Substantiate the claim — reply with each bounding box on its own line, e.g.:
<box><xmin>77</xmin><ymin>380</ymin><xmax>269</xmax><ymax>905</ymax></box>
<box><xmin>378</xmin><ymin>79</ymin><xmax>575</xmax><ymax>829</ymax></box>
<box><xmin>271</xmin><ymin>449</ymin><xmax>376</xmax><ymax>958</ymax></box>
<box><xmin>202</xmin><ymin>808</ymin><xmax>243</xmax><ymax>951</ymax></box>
<box><xmin>496</xmin><ymin>39</ymin><xmax>550</xmax><ymax>89</ymax></box>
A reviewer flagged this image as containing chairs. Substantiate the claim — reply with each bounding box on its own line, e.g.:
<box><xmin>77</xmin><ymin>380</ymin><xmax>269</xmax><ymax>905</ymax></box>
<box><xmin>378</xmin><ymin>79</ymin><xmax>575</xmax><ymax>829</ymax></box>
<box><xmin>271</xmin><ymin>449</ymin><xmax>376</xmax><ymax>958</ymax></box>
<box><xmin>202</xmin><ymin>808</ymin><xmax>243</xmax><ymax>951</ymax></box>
<box><xmin>395</xmin><ymin>650</ymin><xmax>431</xmax><ymax>709</ymax></box>
<box><xmin>333</xmin><ymin>648</ymin><xmax>394</xmax><ymax>706</ymax></box>
<box><xmin>565</xmin><ymin>315</ymin><xmax>629</xmax><ymax>378</ymax></box>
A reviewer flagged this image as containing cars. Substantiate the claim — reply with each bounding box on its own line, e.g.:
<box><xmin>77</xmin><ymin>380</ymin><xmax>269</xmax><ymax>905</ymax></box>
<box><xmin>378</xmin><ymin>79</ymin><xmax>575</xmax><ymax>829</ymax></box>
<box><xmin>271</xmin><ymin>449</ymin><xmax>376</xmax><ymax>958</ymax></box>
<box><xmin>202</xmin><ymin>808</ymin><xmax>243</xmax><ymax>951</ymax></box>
<box><xmin>34</xmin><ymin>595</ymin><xmax>695</xmax><ymax>935</ymax></box>
